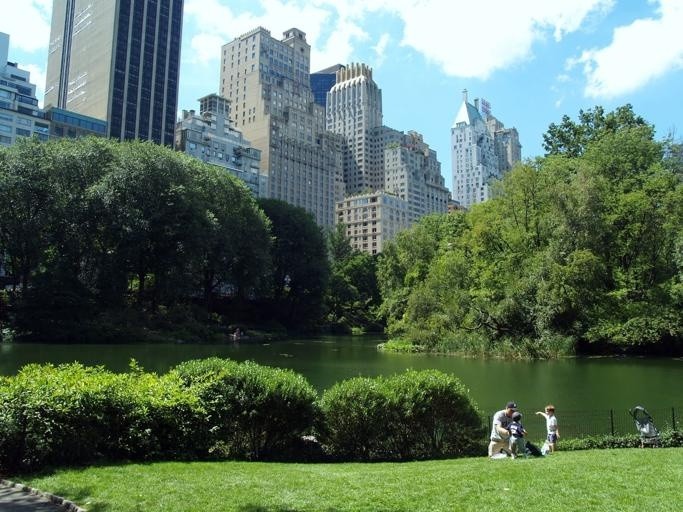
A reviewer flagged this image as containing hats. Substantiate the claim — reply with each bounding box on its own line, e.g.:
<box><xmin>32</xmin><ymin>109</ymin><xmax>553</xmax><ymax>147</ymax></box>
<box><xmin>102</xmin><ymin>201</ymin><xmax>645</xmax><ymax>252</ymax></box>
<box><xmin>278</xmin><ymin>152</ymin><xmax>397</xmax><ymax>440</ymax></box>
<box><xmin>508</xmin><ymin>403</ymin><xmax>518</xmax><ymax>411</ymax></box>
<box><xmin>512</xmin><ymin>412</ymin><xmax>522</xmax><ymax>421</ymax></box>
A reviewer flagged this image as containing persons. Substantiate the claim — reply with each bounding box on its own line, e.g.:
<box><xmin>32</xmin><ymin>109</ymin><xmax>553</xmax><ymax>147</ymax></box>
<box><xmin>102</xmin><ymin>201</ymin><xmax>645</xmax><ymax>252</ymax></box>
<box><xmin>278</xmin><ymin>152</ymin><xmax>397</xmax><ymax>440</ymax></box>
<box><xmin>535</xmin><ymin>405</ymin><xmax>560</xmax><ymax>453</ymax></box>
<box><xmin>488</xmin><ymin>403</ymin><xmax>517</xmax><ymax>458</ymax></box>
<box><xmin>506</xmin><ymin>412</ymin><xmax>527</xmax><ymax>459</ymax></box>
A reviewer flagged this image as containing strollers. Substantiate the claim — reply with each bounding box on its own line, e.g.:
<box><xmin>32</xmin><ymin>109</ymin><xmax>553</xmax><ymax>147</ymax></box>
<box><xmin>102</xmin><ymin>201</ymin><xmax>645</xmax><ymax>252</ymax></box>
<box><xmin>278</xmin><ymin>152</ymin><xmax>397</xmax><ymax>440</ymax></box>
<box><xmin>628</xmin><ymin>406</ymin><xmax>661</xmax><ymax>448</ymax></box>
